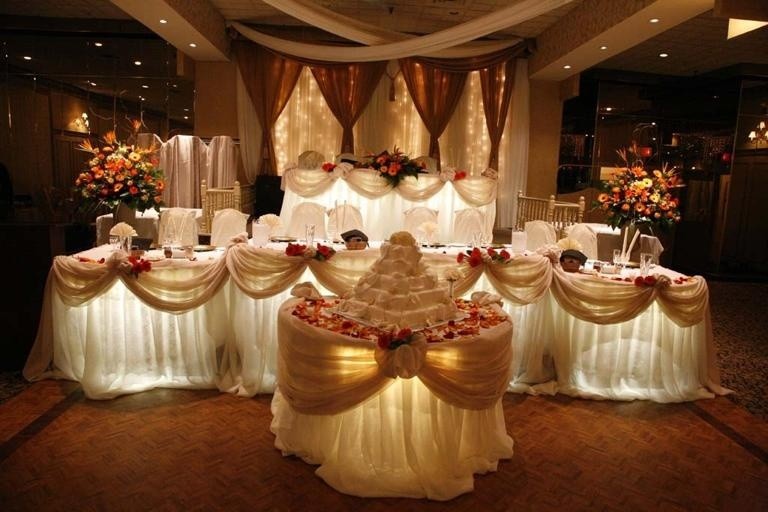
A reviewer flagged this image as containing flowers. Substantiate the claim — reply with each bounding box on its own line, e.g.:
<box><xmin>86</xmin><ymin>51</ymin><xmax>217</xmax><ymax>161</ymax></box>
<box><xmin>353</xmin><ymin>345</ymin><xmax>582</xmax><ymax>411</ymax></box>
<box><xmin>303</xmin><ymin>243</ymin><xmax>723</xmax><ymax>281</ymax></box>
<box><xmin>357</xmin><ymin>144</ymin><xmax>429</xmax><ymax>190</ymax></box>
<box><xmin>322</xmin><ymin>163</ymin><xmax>337</xmax><ymax>172</ymax></box>
<box><xmin>66</xmin><ymin>119</ymin><xmax>170</xmax><ymax>230</ymax></box>
<box><xmin>590</xmin><ymin>138</ymin><xmax>695</xmax><ymax>239</ymax></box>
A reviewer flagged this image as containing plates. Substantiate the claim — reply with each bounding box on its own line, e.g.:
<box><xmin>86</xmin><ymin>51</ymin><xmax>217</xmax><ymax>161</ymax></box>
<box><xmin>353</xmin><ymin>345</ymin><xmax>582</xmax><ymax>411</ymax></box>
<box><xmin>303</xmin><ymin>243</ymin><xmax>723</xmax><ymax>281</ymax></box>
<box><xmin>270</xmin><ymin>235</ymin><xmax>295</xmax><ymax>242</ymax></box>
<box><xmin>479</xmin><ymin>242</ymin><xmax>503</xmax><ymax>249</ymax></box>
<box><xmin>143</xmin><ymin>254</ymin><xmax>164</xmax><ymax>261</ymax></box>
<box><xmin>580</xmin><ymin>269</ymin><xmax>596</xmax><ymax>274</ymax></box>
<box><xmin>192</xmin><ymin>245</ymin><xmax>217</xmax><ymax>252</ymax></box>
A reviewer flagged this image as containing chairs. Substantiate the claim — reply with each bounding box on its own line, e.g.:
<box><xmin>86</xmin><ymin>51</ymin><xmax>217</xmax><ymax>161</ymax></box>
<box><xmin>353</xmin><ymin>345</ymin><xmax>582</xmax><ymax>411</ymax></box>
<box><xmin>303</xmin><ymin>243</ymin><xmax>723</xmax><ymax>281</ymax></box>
<box><xmin>414</xmin><ymin>156</ymin><xmax>437</xmax><ymax>174</ymax></box>
<box><xmin>335</xmin><ymin>153</ymin><xmax>354</xmax><ymax>167</ymax></box>
<box><xmin>298</xmin><ymin>150</ymin><xmax>325</xmax><ymax>170</ymax></box>
<box><xmin>155</xmin><ymin>201</ymin><xmax>598</xmax><ymax>262</ymax></box>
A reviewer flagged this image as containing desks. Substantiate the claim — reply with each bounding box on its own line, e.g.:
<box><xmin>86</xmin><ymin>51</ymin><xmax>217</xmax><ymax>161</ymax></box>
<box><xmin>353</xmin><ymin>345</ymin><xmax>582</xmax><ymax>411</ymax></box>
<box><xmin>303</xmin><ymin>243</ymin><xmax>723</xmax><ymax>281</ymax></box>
<box><xmin>278</xmin><ymin>167</ymin><xmax>499</xmax><ymax>244</ymax></box>
<box><xmin>269</xmin><ymin>291</ymin><xmax>520</xmax><ymax>500</ymax></box>
<box><xmin>30</xmin><ymin>239</ymin><xmax>707</xmax><ymax>402</ymax></box>
<box><xmin>51</xmin><ymin>166</ymin><xmax>710</xmax><ymax>500</ymax></box>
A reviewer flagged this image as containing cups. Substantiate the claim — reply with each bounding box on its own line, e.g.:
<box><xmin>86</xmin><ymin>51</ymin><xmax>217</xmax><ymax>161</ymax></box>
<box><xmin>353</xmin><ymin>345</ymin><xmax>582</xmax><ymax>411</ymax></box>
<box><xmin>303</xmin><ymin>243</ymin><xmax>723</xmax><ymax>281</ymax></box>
<box><xmin>163</xmin><ymin>239</ymin><xmax>173</xmax><ymax>259</ymax></box>
<box><xmin>593</xmin><ymin>262</ymin><xmax>600</xmax><ymax>271</ymax></box>
<box><xmin>305</xmin><ymin>224</ymin><xmax>315</xmax><ymax>244</ymax></box>
<box><xmin>639</xmin><ymin>252</ymin><xmax>653</xmax><ymax>278</ymax></box>
<box><xmin>109</xmin><ymin>237</ymin><xmax>119</xmax><ymax>252</ymax></box>
<box><xmin>511</xmin><ymin>228</ymin><xmax>528</xmax><ymax>251</ymax></box>
<box><xmin>612</xmin><ymin>249</ymin><xmax>625</xmax><ymax>274</ymax></box>
<box><xmin>473</xmin><ymin>232</ymin><xmax>482</xmax><ymax>248</ymax></box>
<box><xmin>183</xmin><ymin>240</ymin><xmax>193</xmax><ymax>258</ymax></box>
<box><xmin>672</xmin><ymin>132</ymin><xmax>679</xmax><ymax>145</ymax></box>
